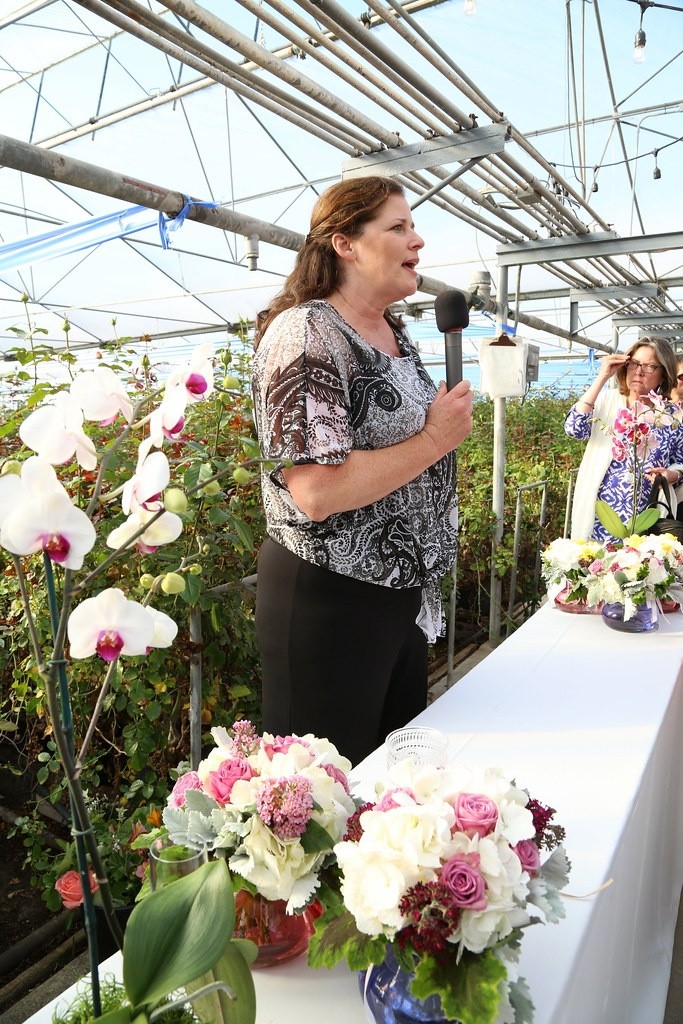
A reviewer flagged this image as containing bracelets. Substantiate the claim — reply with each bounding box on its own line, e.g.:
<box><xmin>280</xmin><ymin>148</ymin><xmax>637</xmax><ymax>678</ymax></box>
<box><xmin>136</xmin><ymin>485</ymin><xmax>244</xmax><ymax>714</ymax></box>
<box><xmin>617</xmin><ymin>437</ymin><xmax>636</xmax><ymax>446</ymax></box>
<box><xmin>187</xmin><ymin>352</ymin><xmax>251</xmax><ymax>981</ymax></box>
<box><xmin>579</xmin><ymin>398</ymin><xmax>596</xmax><ymax>410</ymax></box>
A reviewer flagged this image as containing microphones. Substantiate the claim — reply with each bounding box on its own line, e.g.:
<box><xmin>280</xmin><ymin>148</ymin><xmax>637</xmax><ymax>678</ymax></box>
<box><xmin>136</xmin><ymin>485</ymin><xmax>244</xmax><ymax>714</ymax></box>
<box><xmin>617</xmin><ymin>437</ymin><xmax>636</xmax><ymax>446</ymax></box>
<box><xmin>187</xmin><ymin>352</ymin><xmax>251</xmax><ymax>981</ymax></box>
<box><xmin>435</xmin><ymin>291</ymin><xmax>469</xmax><ymax>391</ymax></box>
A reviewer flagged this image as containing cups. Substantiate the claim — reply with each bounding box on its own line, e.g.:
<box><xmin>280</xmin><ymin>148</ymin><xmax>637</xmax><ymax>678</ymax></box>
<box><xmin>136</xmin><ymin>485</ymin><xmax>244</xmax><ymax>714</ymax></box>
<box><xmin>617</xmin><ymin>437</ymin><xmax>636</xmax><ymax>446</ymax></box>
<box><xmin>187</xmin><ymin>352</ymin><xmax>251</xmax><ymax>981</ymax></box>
<box><xmin>151</xmin><ymin>831</ymin><xmax>209</xmax><ymax>892</ymax></box>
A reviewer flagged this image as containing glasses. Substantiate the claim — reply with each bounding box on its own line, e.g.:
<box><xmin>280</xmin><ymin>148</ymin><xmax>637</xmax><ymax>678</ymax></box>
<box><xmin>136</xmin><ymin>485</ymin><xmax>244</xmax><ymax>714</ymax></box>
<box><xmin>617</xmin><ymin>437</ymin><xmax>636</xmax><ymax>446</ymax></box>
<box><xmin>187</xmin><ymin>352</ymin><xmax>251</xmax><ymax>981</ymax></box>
<box><xmin>624</xmin><ymin>360</ymin><xmax>663</xmax><ymax>374</ymax></box>
<box><xmin>676</xmin><ymin>374</ymin><xmax>683</xmax><ymax>381</ymax></box>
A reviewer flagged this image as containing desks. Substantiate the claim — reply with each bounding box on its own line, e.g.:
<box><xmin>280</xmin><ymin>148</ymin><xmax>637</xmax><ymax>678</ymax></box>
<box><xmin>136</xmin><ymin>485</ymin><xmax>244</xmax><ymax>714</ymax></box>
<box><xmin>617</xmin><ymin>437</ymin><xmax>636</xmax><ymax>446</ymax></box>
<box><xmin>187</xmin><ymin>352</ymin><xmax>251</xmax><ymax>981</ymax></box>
<box><xmin>24</xmin><ymin>584</ymin><xmax>683</xmax><ymax>1024</ymax></box>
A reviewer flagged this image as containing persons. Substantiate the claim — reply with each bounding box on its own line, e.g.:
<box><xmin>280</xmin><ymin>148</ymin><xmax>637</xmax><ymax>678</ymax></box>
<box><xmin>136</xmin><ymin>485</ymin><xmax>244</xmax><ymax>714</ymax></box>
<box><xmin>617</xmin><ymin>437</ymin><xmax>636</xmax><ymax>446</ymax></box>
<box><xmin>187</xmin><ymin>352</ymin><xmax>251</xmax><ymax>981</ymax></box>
<box><xmin>252</xmin><ymin>176</ymin><xmax>475</xmax><ymax>770</ymax></box>
<box><xmin>560</xmin><ymin>335</ymin><xmax>683</xmax><ymax>544</ymax></box>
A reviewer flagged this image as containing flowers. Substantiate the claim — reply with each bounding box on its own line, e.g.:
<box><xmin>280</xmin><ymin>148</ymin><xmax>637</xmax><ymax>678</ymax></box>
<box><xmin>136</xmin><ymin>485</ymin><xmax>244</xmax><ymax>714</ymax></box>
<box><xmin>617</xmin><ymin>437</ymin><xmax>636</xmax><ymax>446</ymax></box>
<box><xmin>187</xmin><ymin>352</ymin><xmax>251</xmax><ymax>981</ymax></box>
<box><xmin>54</xmin><ymin>724</ymin><xmax>571</xmax><ymax>1024</ymax></box>
<box><xmin>539</xmin><ymin>534</ymin><xmax>683</xmax><ymax>624</ymax></box>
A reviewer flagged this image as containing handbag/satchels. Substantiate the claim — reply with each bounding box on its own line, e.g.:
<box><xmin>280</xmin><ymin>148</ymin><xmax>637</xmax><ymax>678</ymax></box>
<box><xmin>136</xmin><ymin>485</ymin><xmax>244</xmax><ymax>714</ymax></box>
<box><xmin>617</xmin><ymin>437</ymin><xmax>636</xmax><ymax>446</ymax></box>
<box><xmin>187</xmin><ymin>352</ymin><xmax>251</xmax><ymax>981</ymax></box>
<box><xmin>639</xmin><ymin>477</ymin><xmax>683</xmax><ymax>546</ymax></box>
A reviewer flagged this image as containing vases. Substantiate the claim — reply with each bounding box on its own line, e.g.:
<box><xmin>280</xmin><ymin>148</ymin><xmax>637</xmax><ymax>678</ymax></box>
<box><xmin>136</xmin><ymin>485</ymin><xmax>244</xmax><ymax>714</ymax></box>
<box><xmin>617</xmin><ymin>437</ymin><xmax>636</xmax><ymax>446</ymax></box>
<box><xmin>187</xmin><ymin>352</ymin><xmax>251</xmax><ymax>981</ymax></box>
<box><xmin>230</xmin><ymin>887</ymin><xmax>316</xmax><ymax>971</ymax></box>
<box><xmin>358</xmin><ymin>938</ymin><xmax>454</xmax><ymax>1024</ymax></box>
<box><xmin>554</xmin><ymin>580</ymin><xmax>603</xmax><ymax>614</ymax></box>
<box><xmin>659</xmin><ymin>598</ymin><xmax>680</xmax><ymax>613</ymax></box>
<box><xmin>601</xmin><ymin>602</ymin><xmax>659</xmax><ymax>633</ymax></box>
